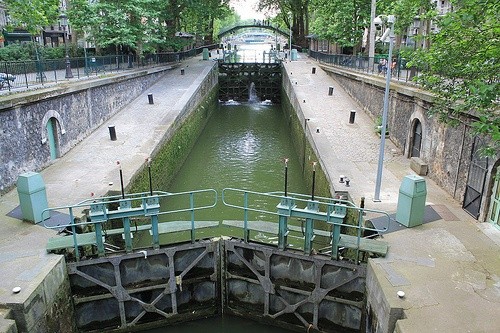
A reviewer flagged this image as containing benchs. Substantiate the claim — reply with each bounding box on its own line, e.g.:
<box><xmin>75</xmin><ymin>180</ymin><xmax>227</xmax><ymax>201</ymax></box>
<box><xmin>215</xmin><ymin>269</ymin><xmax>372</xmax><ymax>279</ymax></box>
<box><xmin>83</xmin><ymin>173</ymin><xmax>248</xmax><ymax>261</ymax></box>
<box><xmin>0</xmin><ymin>72</ymin><xmax>16</xmax><ymax>90</ymax></box>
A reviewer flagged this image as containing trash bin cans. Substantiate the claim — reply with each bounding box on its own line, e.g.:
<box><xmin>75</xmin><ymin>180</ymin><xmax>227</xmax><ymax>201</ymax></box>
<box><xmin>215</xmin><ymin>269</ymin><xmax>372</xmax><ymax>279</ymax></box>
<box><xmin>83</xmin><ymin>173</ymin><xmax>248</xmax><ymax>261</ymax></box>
<box><xmin>203</xmin><ymin>47</ymin><xmax>209</xmax><ymax>60</ymax></box>
<box><xmin>290</xmin><ymin>50</ymin><xmax>297</xmax><ymax>61</ymax></box>
<box><xmin>395</xmin><ymin>174</ymin><xmax>427</xmax><ymax>228</ymax></box>
<box><xmin>16</xmin><ymin>171</ymin><xmax>50</xmax><ymax>225</ymax></box>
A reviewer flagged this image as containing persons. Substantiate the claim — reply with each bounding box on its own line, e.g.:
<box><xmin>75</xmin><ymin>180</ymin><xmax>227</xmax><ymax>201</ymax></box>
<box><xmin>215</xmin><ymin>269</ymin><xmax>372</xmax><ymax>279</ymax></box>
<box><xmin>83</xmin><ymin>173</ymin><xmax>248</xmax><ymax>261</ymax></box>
<box><xmin>251</xmin><ymin>17</ymin><xmax>270</xmax><ymax>27</ymax></box>
<box><xmin>341</xmin><ymin>52</ymin><xmax>397</xmax><ymax>76</ymax></box>
